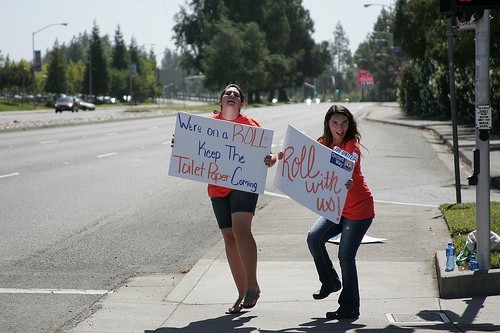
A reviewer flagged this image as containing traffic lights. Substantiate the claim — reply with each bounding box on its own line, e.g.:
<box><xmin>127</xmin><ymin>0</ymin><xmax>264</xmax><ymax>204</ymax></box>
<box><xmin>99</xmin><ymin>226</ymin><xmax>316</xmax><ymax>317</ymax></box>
<box><xmin>336</xmin><ymin>89</ymin><xmax>340</xmax><ymax>93</ymax></box>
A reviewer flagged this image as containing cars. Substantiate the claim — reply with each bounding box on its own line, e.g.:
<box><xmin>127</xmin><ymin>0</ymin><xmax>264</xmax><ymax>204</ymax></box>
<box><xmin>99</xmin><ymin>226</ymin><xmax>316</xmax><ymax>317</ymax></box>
<box><xmin>55</xmin><ymin>96</ymin><xmax>96</xmax><ymax>113</ymax></box>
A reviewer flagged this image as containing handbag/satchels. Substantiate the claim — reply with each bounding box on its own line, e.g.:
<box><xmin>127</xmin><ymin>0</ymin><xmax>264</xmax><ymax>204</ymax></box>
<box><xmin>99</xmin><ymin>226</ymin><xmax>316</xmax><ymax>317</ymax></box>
<box><xmin>456</xmin><ymin>230</ymin><xmax>500</xmax><ymax>265</ymax></box>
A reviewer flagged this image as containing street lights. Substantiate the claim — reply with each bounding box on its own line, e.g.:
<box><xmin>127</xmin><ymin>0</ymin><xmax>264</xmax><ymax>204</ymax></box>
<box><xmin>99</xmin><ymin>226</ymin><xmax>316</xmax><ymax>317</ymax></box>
<box><xmin>32</xmin><ymin>23</ymin><xmax>67</xmax><ymax>109</ymax></box>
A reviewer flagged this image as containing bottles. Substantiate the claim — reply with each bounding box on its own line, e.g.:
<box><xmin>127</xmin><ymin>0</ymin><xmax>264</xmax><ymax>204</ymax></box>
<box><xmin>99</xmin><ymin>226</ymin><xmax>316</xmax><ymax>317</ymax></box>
<box><xmin>445</xmin><ymin>242</ymin><xmax>455</xmax><ymax>271</ymax></box>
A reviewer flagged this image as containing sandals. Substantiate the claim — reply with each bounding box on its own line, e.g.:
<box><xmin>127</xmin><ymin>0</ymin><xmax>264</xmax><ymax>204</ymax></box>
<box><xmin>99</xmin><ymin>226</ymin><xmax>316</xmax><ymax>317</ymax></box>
<box><xmin>226</xmin><ymin>299</ymin><xmax>245</xmax><ymax>314</ymax></box>
<box><xmin>243</xmin><ymin>285</ymin><xmax>260</xmax><ymax>309</ymax></box>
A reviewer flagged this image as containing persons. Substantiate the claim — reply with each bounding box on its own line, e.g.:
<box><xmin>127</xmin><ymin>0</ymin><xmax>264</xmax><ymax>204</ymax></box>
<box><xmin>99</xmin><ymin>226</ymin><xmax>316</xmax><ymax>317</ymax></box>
<box><xmin>278</xmin><ymin>105</ymin><xmax>375</xmax><ymax>319</ymax></box>
<box><xmin>170</xmin><ymin>84</ymin><xmax>276</xmax><ymax>313</ymax></box>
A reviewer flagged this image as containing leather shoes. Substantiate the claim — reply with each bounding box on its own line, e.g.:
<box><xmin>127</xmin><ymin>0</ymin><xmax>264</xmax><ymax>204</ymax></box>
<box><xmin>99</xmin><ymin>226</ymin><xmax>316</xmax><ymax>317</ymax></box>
<box><xmin>326</xmin><ymin>305</ymin><xmax>360</xmax><ymax>319</ymax></box>
<box><xmin>313</xmin><ymin>279</ymin><xmax>341</xmax><ymax>300</ymax></box>
